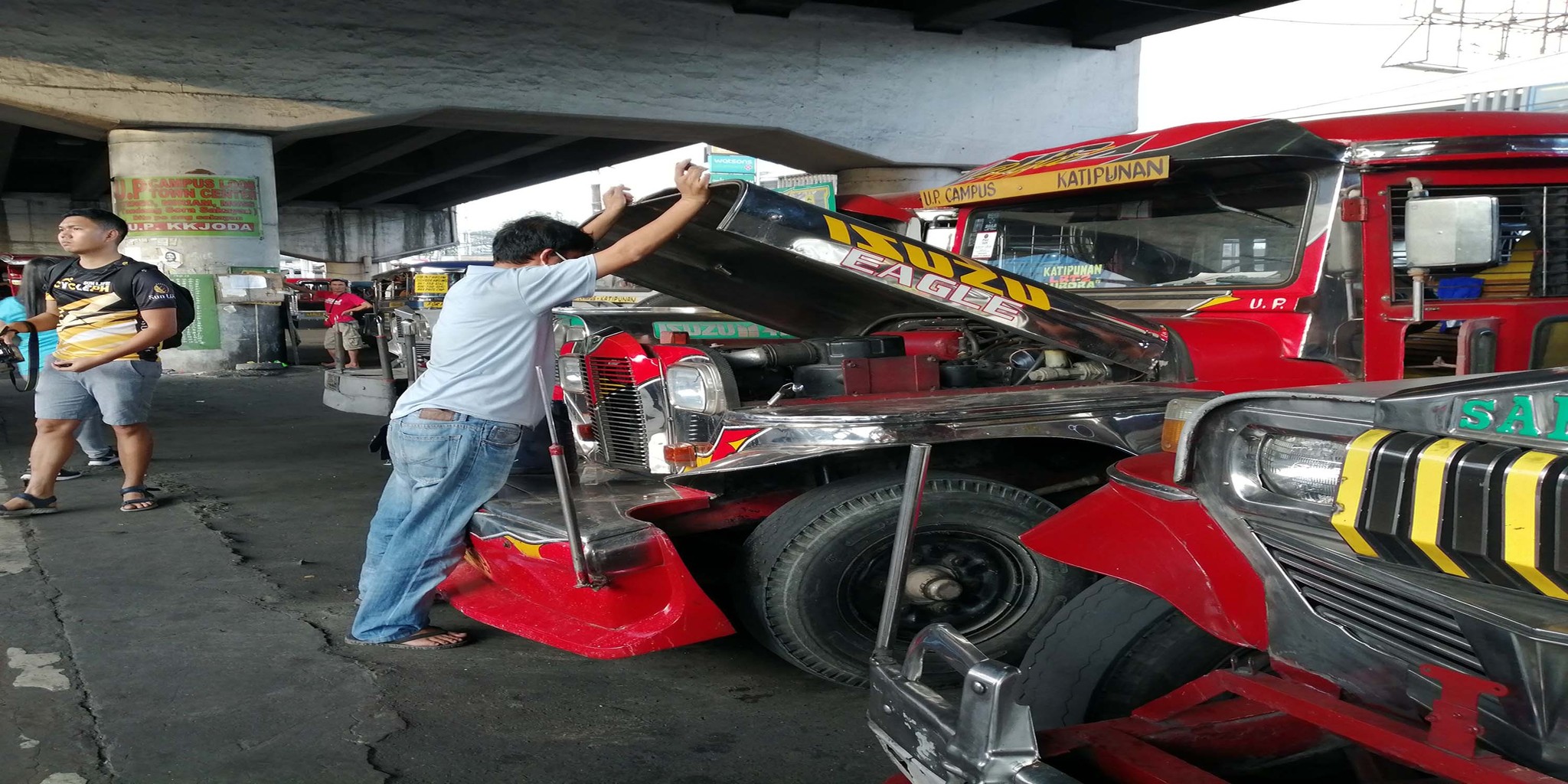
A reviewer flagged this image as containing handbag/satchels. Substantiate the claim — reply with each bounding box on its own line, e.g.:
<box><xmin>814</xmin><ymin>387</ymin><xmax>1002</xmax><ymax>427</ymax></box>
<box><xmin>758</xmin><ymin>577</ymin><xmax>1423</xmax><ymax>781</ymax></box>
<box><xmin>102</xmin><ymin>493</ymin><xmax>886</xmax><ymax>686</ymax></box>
<box><xmin>325</xmin><ymin>318</ymin><xmax>332</xmax><ymax>327</ymax></box>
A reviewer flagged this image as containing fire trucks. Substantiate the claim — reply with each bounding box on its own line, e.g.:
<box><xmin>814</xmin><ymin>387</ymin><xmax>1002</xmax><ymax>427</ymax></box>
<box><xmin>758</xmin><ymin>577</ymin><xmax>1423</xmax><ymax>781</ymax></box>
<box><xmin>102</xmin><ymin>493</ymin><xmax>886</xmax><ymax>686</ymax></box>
<box><xmin>865</xmin><ymin>367</ymin><xmax>1567</xmax><ymax>784</ymax></box>
<box><xmin>279</xmin><ymin>105</ymin><xmax>1565</xmax><ymax>694</ymax></box>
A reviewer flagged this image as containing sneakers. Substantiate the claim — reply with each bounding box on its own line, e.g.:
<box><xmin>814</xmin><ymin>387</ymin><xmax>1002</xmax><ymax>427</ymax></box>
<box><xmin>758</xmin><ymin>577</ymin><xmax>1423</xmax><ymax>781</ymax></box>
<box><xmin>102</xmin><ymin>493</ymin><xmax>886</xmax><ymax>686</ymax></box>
<box><xmin>20</xmin><ymin>465</ymin><xmax>82</xmax><ymax>482</ymax></box>
<box><xmin>88</xmin><ymin>446</ymin><xmax>119</xmax><ymax>465</ymax></box>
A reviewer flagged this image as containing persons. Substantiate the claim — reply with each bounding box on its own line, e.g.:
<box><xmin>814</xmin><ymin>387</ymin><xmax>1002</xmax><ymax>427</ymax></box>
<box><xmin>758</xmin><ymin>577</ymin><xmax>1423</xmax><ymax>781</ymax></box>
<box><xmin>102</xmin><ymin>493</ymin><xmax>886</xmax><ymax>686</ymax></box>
<box><xmin>0</xmin><ymin>257</ymin><xmax>119</xmax><ymax>482</ymax></box>
<box><xmin>345</xmin><ymin>158</ymin><xmax>710</xmax><ymax>650</ymax></box>
<box><xmin>282</xmin><ymin>276</ymin><xmax>373</xmax><ymax>368</ymax></box>
<box><xmin>0</xmin><ymin>207</ymin><xmax>178</xmax><ymax>515</ymax></box>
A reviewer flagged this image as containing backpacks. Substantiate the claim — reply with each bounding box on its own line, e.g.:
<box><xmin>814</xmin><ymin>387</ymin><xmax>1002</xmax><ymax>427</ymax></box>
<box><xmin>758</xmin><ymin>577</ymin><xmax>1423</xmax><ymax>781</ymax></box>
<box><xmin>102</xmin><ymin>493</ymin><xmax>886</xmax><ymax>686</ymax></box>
<box><xmin>48</xmin><ymin>257</ymin><xmax>195</xmax><ymax>349</ymax></box>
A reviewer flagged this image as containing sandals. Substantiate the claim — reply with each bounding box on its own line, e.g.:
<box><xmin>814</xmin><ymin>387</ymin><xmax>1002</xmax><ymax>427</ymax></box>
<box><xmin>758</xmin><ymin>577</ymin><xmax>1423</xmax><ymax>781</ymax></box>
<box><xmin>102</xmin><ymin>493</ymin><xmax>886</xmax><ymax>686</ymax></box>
<box><xmin>0</xmin><ymin>493</ymin><xmax>59</xmax><ymax>515</ymax></box>
<box><xmin>120</xmin><ymin>484</ymin><xmax>161</xmax><ymax>511</ymax></box>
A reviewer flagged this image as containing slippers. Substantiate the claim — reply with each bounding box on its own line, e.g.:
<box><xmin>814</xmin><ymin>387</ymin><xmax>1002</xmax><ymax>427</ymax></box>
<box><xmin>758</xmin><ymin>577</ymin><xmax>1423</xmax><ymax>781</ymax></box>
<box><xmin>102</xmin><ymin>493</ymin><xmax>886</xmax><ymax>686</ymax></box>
<box><xmin>354</xmin><ymin>598</ymin><xmax>363</xmax><ymax>605</ymax></box>
<box><xmin>344</xmin><ymin>626</ymin><xmax>468</xmax><ymax>650</ymax></box>
